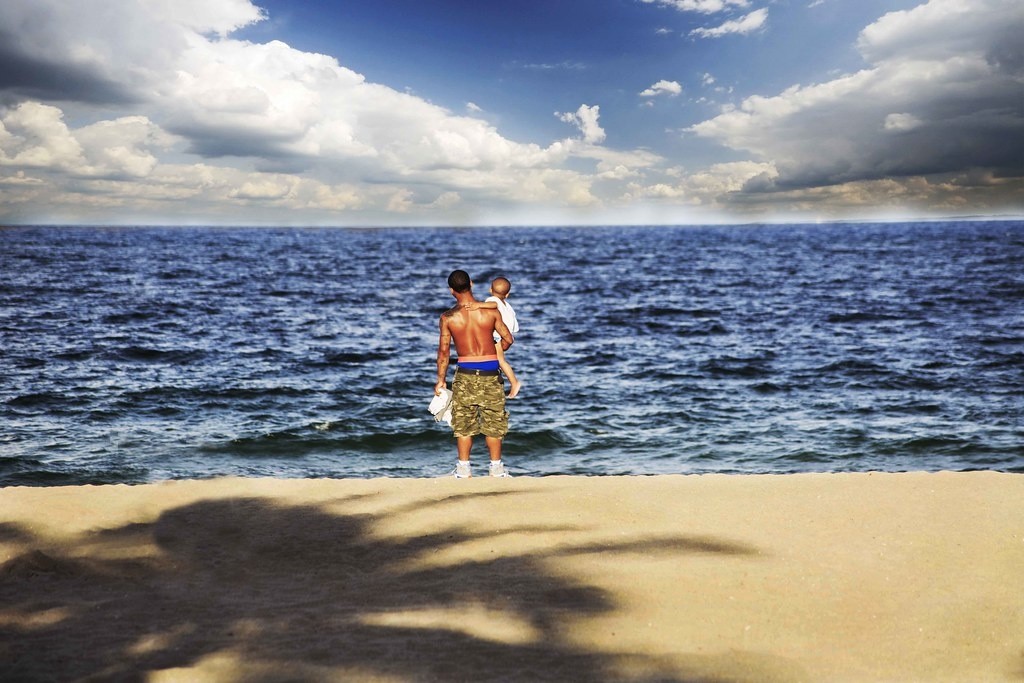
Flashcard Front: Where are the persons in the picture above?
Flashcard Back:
[466,277,521,396]
[434,270,514,479]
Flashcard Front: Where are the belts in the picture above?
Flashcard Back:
[458,368,498,376]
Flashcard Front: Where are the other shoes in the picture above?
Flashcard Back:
[452,464,472,478]
[489,463,512,477]
